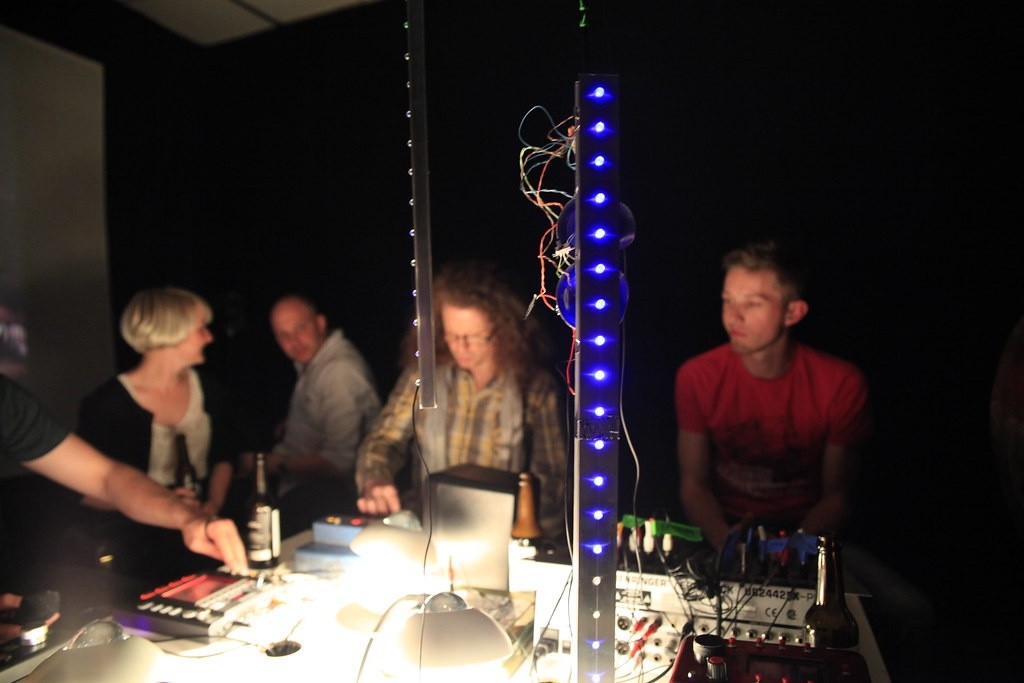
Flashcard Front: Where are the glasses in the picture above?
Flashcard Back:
[443,322,499,346]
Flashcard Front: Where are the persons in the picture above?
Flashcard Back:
[353,264,570,540]
[0,372,248,644]
[78,286,236,613]
[243,296,380,536]
[676,245,934,683]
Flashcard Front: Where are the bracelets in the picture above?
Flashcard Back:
[279,458,288,472]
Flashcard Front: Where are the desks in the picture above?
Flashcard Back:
[1,511,890,683]
